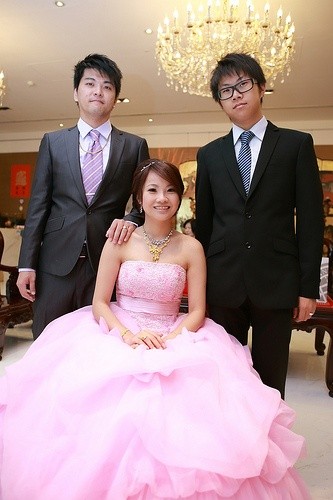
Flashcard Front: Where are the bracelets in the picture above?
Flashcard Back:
[121,329,129,337]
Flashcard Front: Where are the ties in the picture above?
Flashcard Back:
[81,130,103,206]
[237,131,255,196]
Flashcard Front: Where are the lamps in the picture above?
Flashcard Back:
[157,0,298,97]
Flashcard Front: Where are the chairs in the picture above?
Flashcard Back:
[0,227,33,361]
[292,237,333,397]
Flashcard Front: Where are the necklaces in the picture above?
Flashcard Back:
[143,226,174,262]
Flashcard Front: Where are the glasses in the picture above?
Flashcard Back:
[217,77,258,101]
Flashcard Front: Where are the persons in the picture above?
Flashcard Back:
[0,159,310,500]
[16,53,150,341]
[183,219,195,236]
[195,53,325,400]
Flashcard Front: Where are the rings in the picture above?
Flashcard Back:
[309,312,313,315]
[123,225,128,230]
[142,336,147,340]
